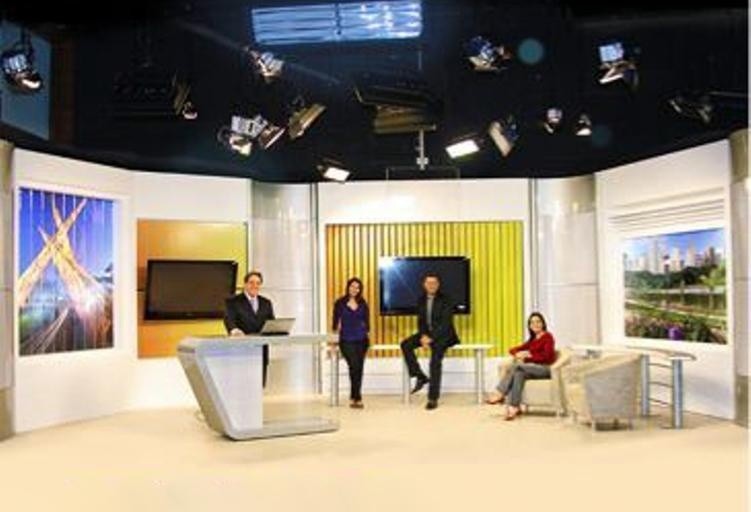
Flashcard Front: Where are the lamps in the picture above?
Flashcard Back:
[444,107,598,161]
[1,26,44,93]
[462,26,513,74]
[217,43,355,185]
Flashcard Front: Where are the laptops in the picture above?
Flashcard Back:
[251,318,294,336]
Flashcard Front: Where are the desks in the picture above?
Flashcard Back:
[324,344,496,408]
[176,333,341,441]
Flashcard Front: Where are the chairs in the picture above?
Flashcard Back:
[561,349,638,433]
[501,346,570,417]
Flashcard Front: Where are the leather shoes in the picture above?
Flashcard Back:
[411,376,429,395]
[426,400,438,409]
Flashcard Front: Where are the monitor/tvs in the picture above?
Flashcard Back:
[145,259,238,319]
[379,256,470,316]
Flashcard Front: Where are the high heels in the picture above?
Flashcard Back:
[504,410,522,420]
[484,394,505,405]
[349,399,357,409]
[358,400,363,408]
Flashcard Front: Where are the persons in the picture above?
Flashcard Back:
[223,271,275,389]
[400,270,462,410]
[485,312,557,420]
[332,276,370,410]
